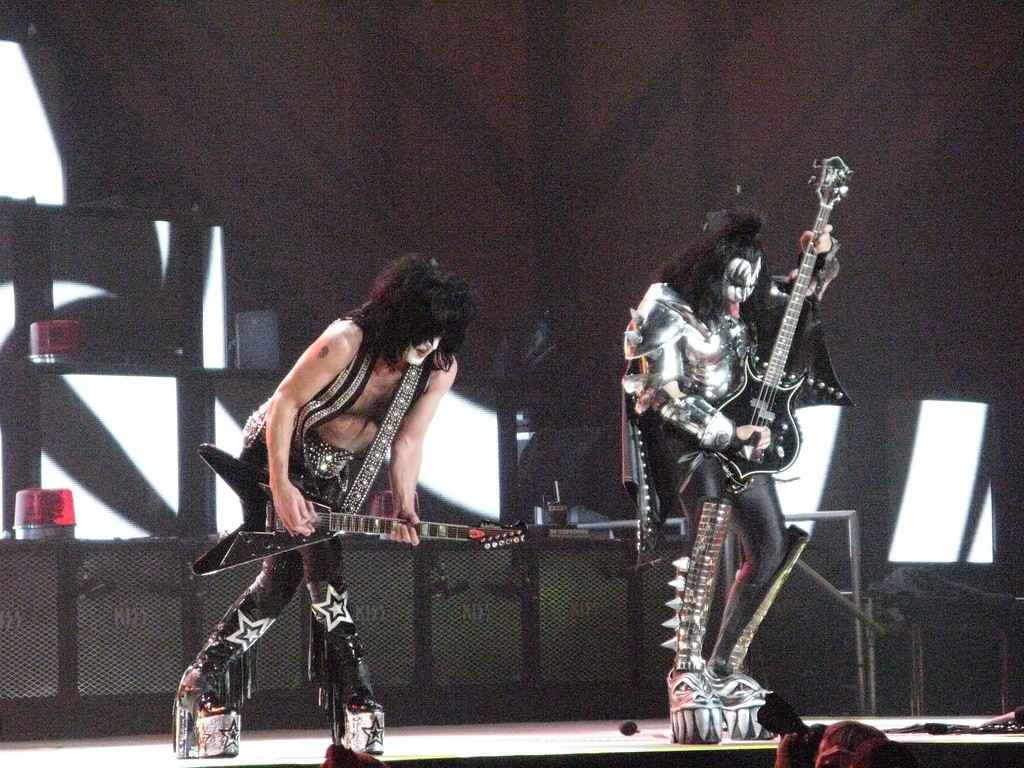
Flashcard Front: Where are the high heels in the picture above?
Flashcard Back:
[172,668,240,760]
[336,681,385,755]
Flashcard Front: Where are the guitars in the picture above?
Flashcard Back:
[703,152,857,483]
[183,438,531,577]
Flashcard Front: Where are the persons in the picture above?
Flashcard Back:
[773,721,919,768]
[171,255,470,759]
[622,208,839,745]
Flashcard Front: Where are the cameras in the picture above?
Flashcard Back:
[757,692,827,768]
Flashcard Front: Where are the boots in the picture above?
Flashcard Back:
[705,524,811,741]
[659,498,733,744]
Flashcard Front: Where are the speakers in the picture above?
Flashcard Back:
[0,529,741,740]
